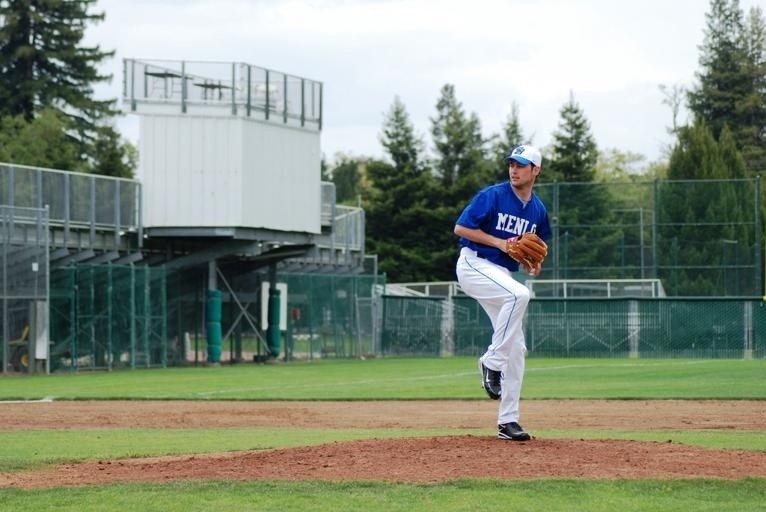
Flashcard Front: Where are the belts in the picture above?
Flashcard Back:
[476,251,487,259]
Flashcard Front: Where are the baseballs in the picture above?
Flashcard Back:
[523,263,535,276]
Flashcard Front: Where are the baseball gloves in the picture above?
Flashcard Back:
[506,232,548,272]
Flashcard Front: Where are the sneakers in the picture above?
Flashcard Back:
[480,357,503,400]
[498,420,531,440]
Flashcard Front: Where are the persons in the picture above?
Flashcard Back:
[451,143,555,444]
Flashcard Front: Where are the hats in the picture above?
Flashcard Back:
[504,144,543,170]
